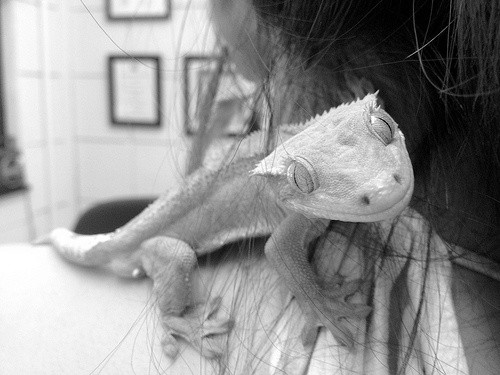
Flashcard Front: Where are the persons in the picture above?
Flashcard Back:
[192,0,498,373]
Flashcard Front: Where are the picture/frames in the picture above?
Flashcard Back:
[108,53,161,127]
[181,54,262,138]
[104,0,174,22]
